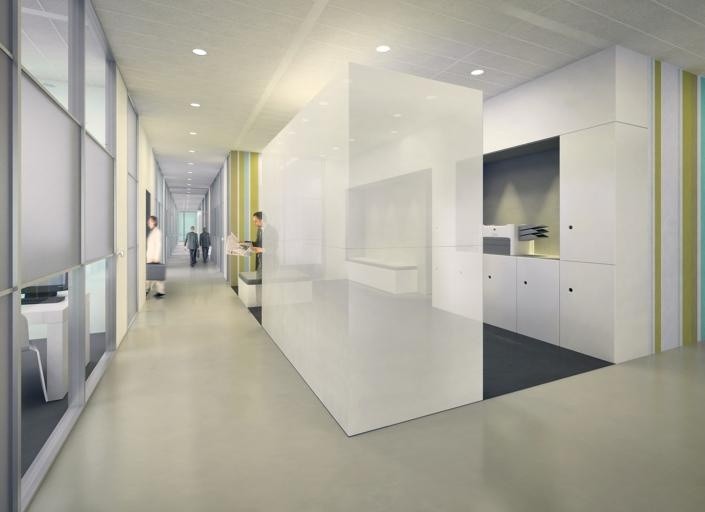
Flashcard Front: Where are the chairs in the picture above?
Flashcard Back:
[20,312,50,403]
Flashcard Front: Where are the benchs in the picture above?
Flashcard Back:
[238,271,314,309]
[348,257,420,296]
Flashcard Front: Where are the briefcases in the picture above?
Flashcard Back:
[146,262,166,281]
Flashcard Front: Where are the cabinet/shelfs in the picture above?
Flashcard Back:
[431,122,655,364]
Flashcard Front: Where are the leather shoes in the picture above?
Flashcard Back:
[154,292,164,297]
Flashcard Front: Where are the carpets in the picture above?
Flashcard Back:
[230,277,615,401]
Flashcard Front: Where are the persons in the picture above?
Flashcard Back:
[183,226,199,267]
[240,212,279,270]
[199,228,211,263]
[146,216,166,296]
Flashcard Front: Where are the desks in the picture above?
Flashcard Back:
[21,288,88,402]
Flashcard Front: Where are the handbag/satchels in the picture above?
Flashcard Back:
[196,248,200,257]
